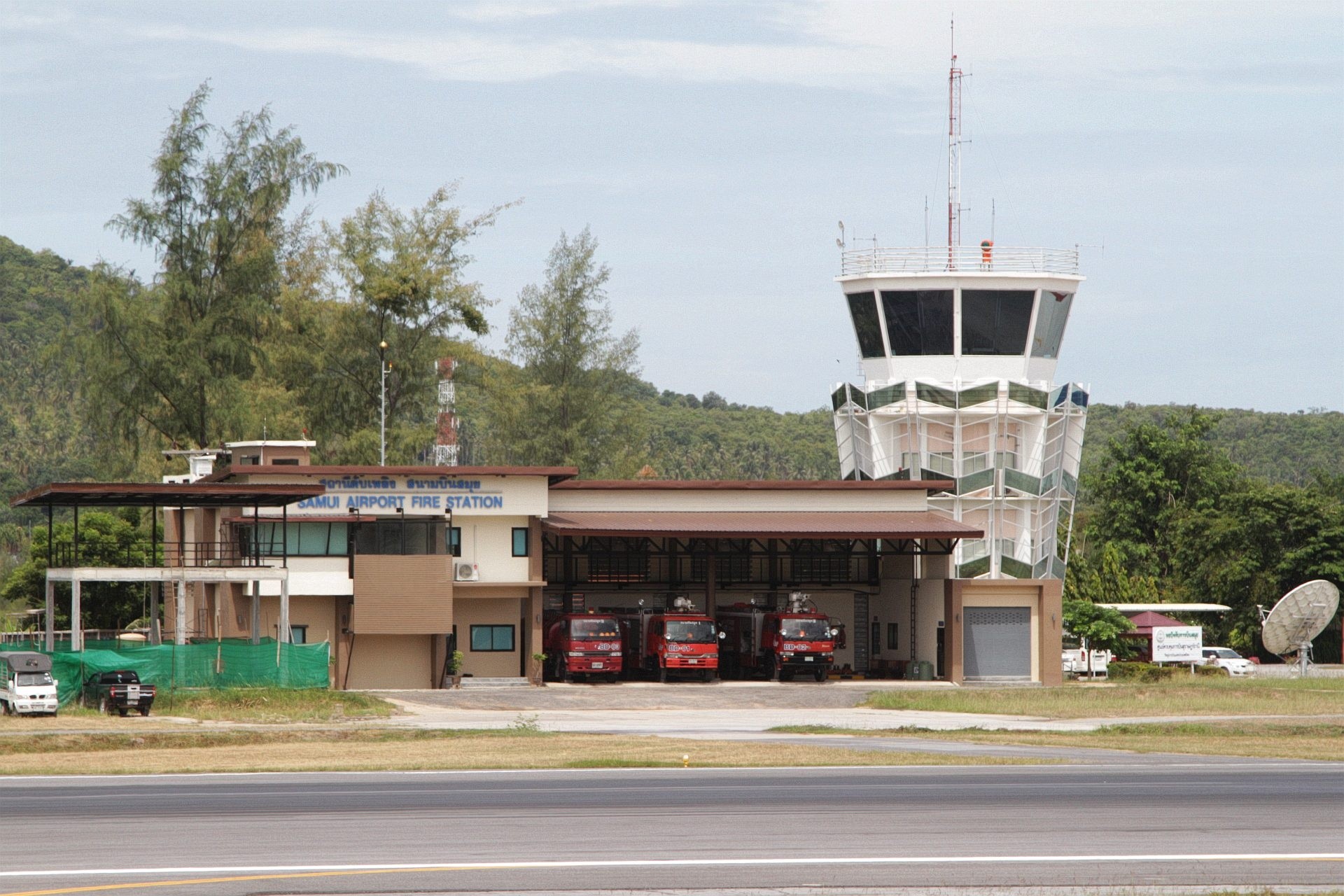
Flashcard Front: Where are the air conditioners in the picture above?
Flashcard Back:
[455,562,479,581]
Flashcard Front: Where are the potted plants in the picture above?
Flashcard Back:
[532,654,548,683]
[448,650,464,685]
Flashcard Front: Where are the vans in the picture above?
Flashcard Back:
[1061,632,1117,673]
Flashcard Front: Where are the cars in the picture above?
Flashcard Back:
[1185,646,1260,678]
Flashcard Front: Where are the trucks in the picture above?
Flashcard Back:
[0,652,60,719]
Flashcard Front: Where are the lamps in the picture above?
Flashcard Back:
[446,508,452,521]
[349,507,359,520]
[396,508,404,519]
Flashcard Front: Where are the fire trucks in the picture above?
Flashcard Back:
[598,597,726,682]
[543,605,622,683]
[712,591,844,682]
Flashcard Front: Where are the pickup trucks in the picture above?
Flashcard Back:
[83,670,156,718]
